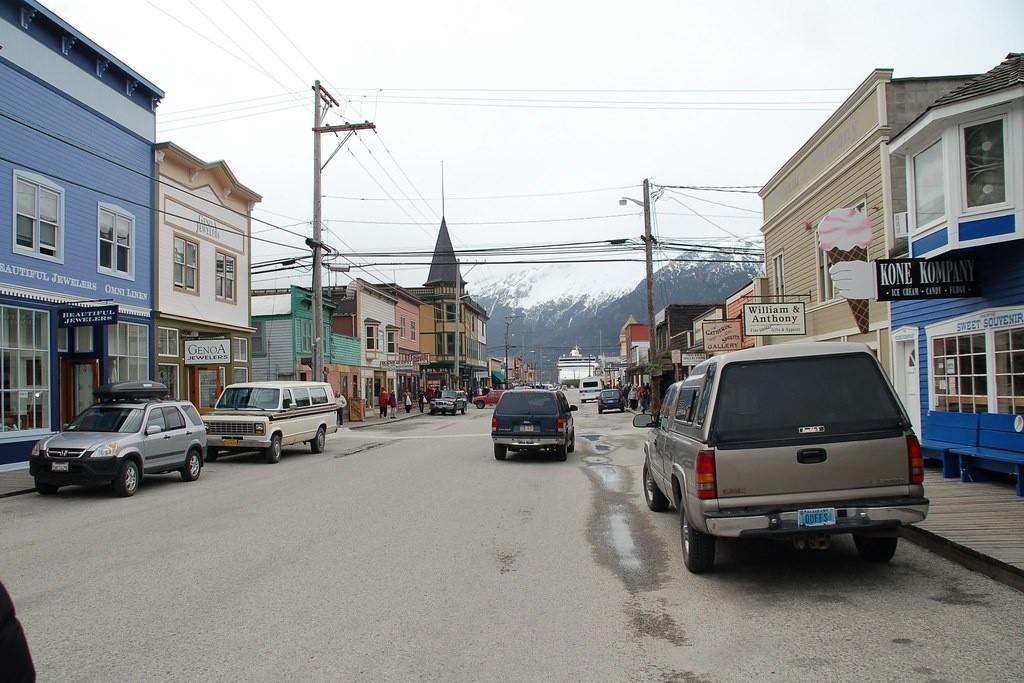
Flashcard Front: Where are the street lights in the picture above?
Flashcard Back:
[609,238,660,424]
[453,293,480,388]
[522,350,535,385]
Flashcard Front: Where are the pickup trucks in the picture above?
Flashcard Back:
[631,338,931,573]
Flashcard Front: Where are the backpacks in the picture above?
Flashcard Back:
[641,392,647,400]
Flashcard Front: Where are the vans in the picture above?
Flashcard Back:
[491,388,577,461]
[579,377,605,403]
[201,380,338,463]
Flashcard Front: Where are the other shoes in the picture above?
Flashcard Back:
[390,417,396,419]
[340,425,343,427]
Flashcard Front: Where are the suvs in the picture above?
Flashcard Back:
[29,380,209,496]
[473,389,508,410]
[429,390,468,415]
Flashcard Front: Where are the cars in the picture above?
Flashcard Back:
[595,388,625,414]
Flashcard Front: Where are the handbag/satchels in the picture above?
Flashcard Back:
[406,395,411,405]
[423,396,427,403]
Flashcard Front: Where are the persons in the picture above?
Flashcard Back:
[622,382,650,414]
[461,386,482,403]
[403,389,412,413]
[482,386,493,396]
[335,392,347,428]
[425,385,440,404]
[605,383,612,389]
[418,388,426,413]
[378,386,389,419]
[388,391,397,419]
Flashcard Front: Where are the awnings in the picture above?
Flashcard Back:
[491,371,512,384]
